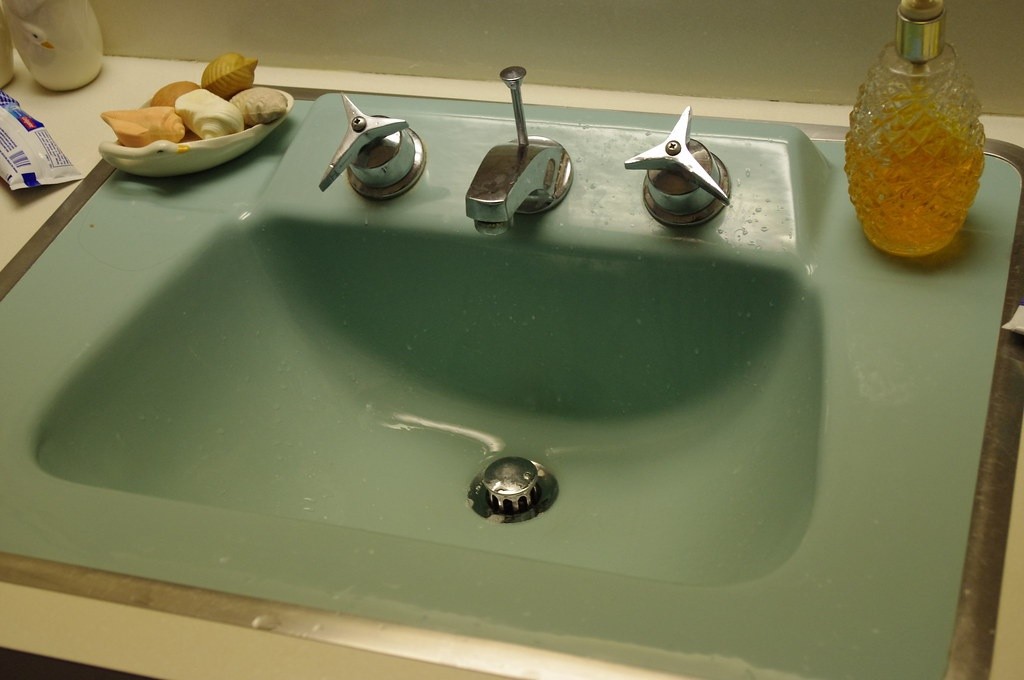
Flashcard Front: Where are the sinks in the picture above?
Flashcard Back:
[30,205,841,593]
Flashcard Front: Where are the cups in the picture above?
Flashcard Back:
[0,9,14,89]
[0,0,103,90]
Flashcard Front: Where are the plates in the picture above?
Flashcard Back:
[98,90,295,175]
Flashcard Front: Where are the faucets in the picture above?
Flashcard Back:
[464,64,574,237]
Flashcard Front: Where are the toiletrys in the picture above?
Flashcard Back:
[842,0,984,262]
[0,87,86,197]
